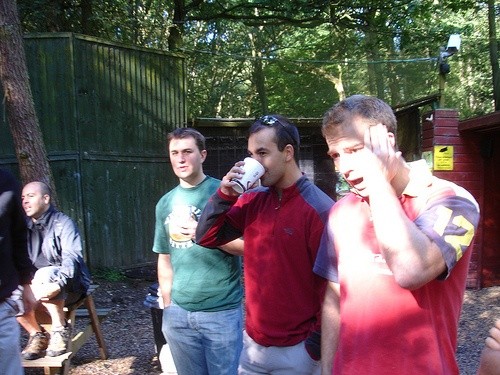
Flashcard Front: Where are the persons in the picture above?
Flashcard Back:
[153,127,244,375]
[10,181,90,361]
[311,94,481,375]
[195,113,338,375]
[0,170,37,375]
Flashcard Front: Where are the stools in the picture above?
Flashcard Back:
[23,285,108,375]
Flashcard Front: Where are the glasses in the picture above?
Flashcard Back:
[254,115,298,144]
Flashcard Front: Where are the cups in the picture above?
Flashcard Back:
[229,156,266,193]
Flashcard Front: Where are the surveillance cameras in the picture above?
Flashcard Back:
[447,35,461,52]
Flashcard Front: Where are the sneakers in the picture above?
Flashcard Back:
[46,317,71,357]
[21,326,50,359]
